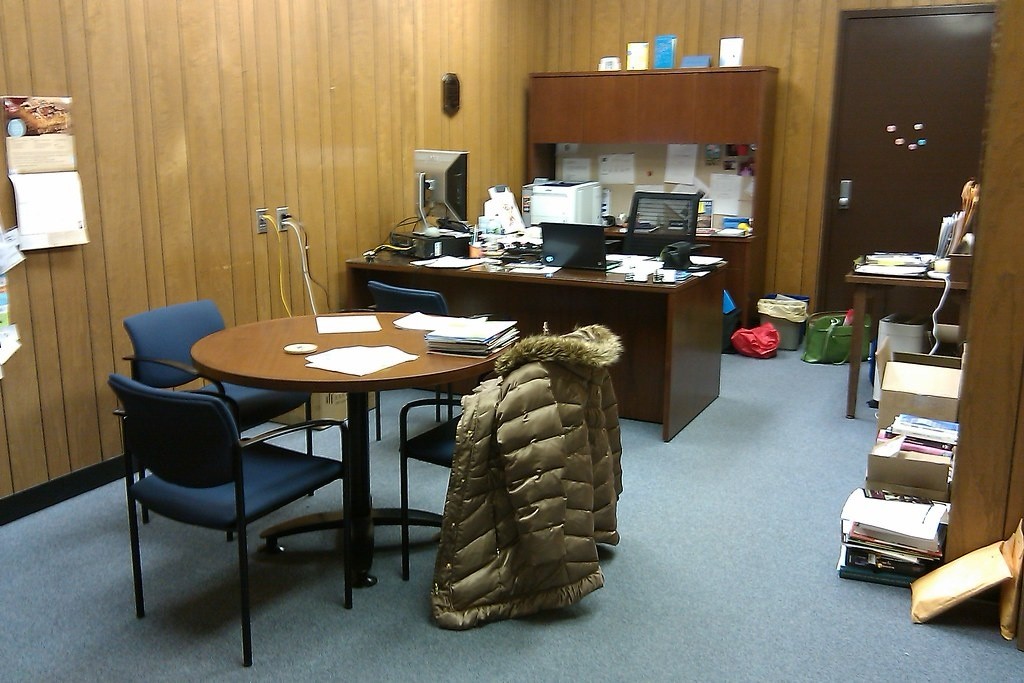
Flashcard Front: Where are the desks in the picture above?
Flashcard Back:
[345,238,728,442]
[844,270,971,419]
[191,312,513,589]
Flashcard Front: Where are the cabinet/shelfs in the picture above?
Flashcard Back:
[526,65,780,329]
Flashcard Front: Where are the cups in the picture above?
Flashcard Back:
[468,242,483,258]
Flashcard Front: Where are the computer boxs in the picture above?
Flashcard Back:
[391,232,473,259]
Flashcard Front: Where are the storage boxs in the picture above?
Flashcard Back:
[865,453,950,502]
[874,336,961,434]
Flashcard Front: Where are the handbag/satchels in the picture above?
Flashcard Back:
[730,322,780,358]
[800,310,871,365]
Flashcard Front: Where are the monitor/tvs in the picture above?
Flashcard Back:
[411,148,472,238]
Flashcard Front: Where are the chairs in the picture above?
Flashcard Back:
[622,191,700,256]
[368,280,448,317]
[121,299,315,497]
[399,324,604,580]
[105,372,355,667]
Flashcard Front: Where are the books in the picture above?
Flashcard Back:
[835,415,960,589]
[427,321,521,358]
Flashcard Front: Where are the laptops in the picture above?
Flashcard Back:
[541,222,623,270]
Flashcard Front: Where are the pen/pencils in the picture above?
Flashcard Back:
[472,224,477,244]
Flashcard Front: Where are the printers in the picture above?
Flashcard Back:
[531,181,603,225]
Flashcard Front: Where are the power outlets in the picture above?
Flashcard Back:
[257,208,268,234]
[277,207,289,233]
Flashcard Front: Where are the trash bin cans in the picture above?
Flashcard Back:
[756,298,807,351]
[764,294,810,344]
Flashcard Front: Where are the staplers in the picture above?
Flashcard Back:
[738,223,754,237]
